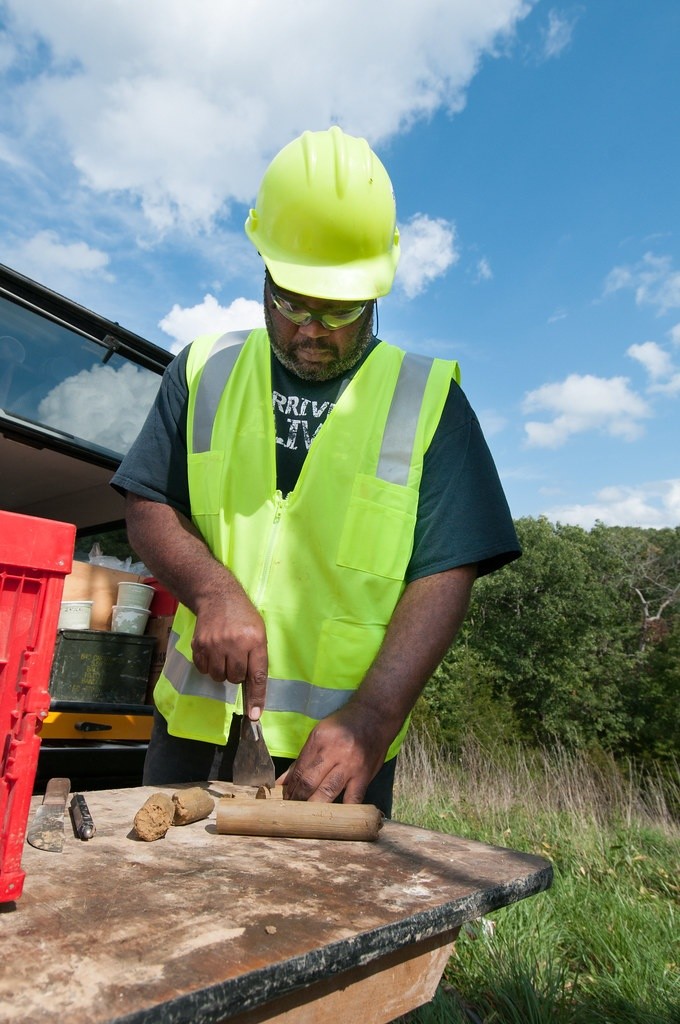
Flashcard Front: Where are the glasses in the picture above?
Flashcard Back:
[265,271,369,329]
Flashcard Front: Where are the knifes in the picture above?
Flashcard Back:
[28,777,72,850]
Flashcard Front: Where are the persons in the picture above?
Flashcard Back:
[109,127,524,820]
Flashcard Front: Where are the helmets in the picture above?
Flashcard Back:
[243,125,402,300]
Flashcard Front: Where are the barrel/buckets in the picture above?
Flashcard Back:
[142,579,179,617]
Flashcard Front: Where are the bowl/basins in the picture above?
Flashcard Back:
[117,582,157,610]
[58,601,94,630]
[111,605,151,635]
